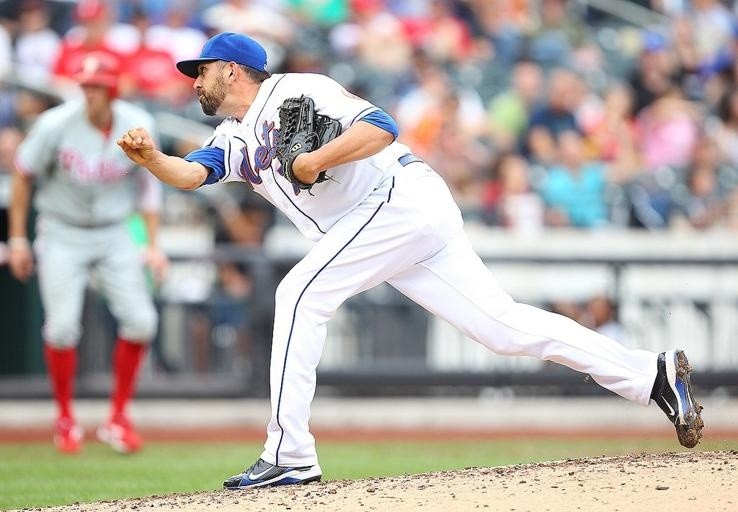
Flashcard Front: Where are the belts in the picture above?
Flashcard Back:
[399,154,423,167]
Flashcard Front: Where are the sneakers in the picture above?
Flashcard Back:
[223,458,323,491]
[655,349,703,449]
[54,417,143,454]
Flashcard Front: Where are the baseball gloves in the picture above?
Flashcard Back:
[273,94,343,194]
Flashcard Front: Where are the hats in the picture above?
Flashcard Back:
[176,31,268,80]
[75,52,120,87]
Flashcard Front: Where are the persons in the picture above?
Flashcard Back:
[116,32,705,492]
[8,51,170,455]
